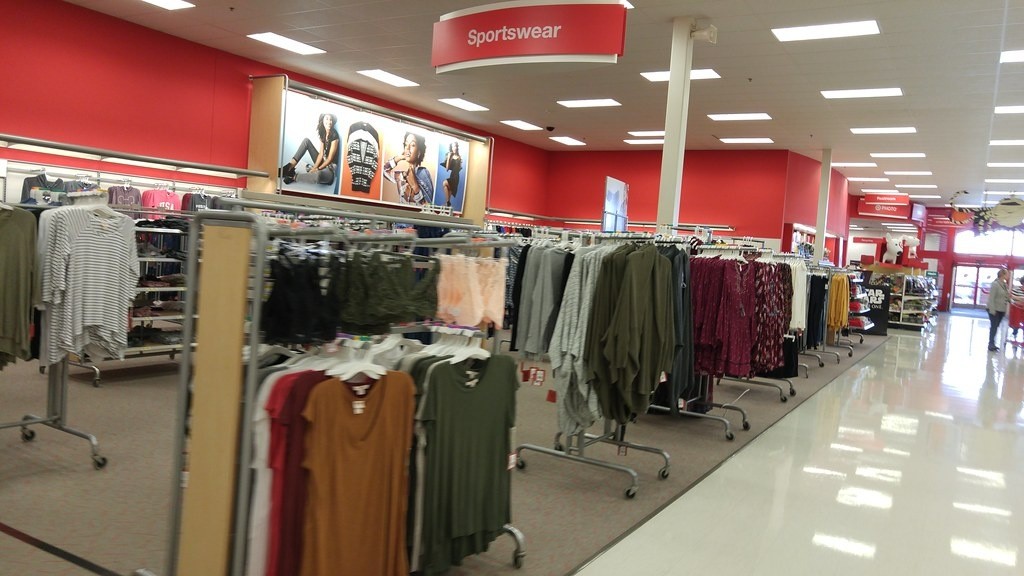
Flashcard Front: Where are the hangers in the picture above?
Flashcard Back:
[490,222,849,275]
[261,327,493,381]
[31,166,234,196]
[0,198,126,222]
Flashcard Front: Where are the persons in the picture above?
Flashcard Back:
[959,274,970,303]
[278,113,340,186]
[440,142,463,210]
[1009,277,1024,338]
[988,269,1011,351]
[383,131,434,206]
[618,184,628,231]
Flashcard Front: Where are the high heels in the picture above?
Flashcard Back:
[306,164,313,172]
[278,163,296,184]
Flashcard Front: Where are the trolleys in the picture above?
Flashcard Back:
[1006,300,1024,354]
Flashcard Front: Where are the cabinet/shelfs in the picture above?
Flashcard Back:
[36,229,198,374]
[844,267,938,337]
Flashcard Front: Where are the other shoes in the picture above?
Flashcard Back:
[988,345,999,352]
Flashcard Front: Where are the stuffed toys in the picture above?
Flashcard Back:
[948,190,1024,236]
[882,233,921,264]
